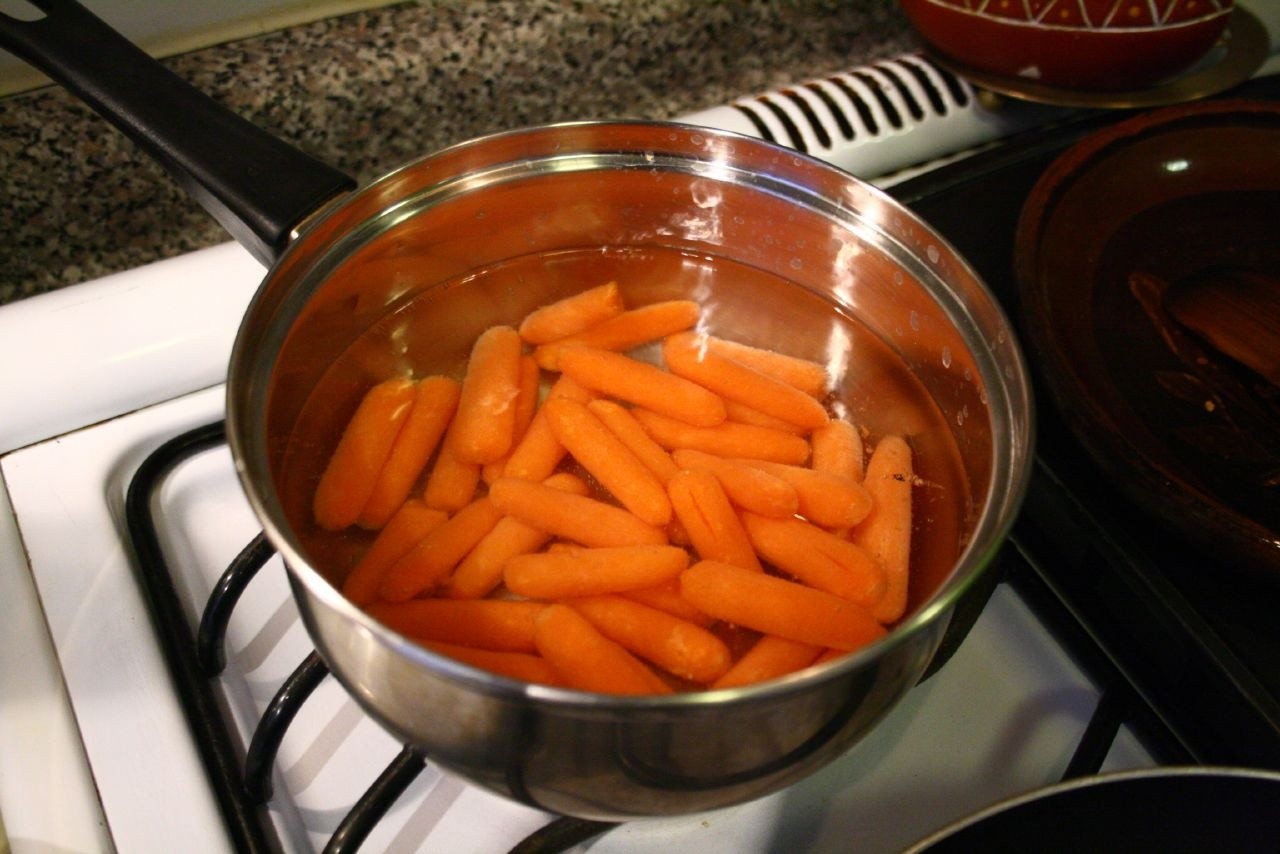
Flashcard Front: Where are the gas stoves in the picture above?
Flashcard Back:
[2,55,1280,853]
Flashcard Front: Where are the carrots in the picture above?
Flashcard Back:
[307,283,912,698]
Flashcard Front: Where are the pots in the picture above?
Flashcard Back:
[896,764,1280,853]
[1015,96,1280,578]
[0,1,1033,822]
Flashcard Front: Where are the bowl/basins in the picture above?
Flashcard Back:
[901,0,1238,89]
